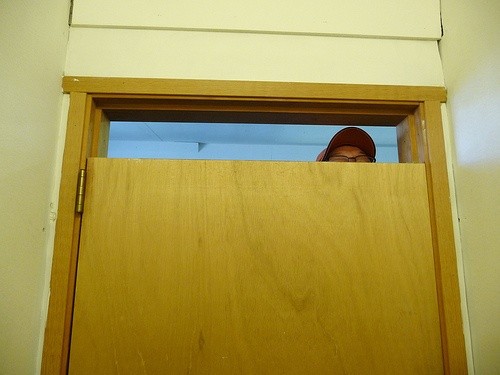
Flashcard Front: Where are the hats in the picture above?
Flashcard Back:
[316,126,376,162]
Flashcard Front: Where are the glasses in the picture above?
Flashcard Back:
[325,154,376,163]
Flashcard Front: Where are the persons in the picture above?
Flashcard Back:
[316,127,377,163]
